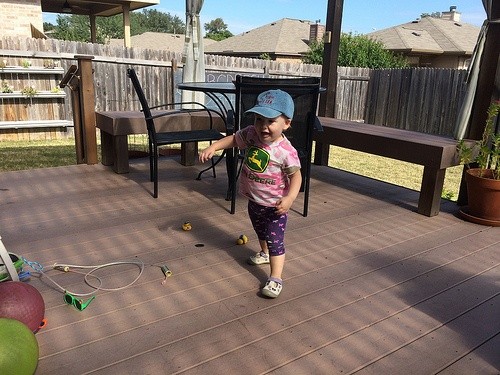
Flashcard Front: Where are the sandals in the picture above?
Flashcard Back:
[261,274,283,297]
[247,250,270,264]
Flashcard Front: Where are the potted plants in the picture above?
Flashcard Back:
[455,103,500,227]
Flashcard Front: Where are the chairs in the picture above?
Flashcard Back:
[127,68,238,201]
[226,75,320,217]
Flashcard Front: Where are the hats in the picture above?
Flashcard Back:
[245,89,295,119]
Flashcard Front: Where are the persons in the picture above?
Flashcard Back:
[198,89,303,298]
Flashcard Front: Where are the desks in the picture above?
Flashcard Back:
[176,82,326,201]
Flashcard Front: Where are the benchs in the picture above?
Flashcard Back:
[94,109,234,175]
[311,116,481,217]
